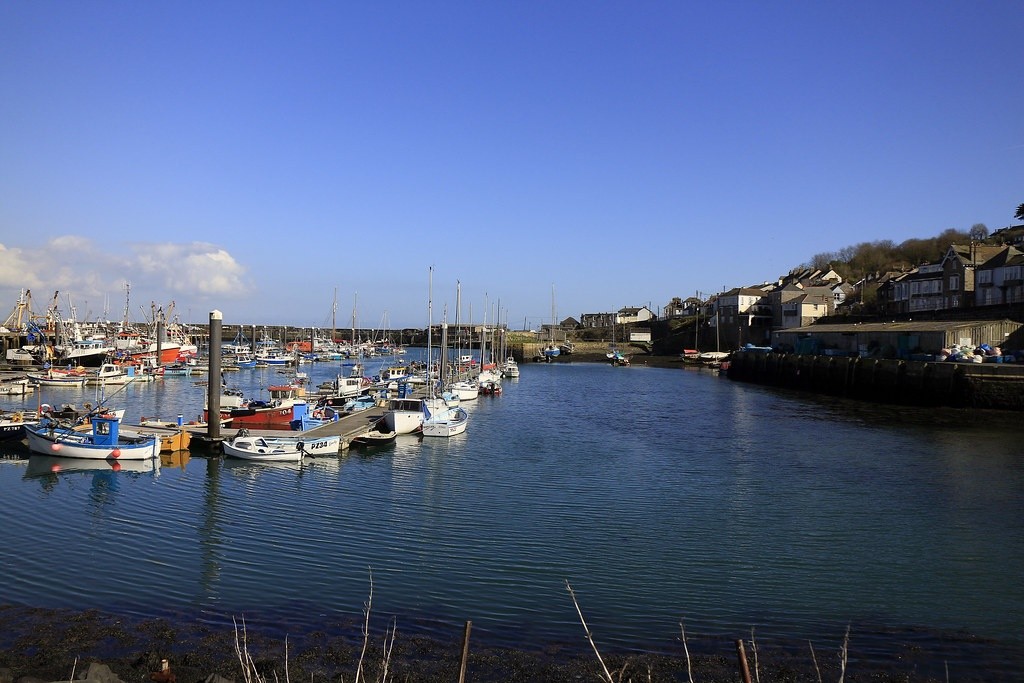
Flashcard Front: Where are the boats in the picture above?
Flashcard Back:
[680,348,730,365]
[605,350,631,367]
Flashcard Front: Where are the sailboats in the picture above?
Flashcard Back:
[1,264,526,470]
[533,281,575,363]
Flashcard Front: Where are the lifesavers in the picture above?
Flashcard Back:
[313,408,324,417]
[98,414,111,417]
[361,377,369,384]
[39,403,51,415]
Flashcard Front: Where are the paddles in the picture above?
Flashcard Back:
[298,446,315,458]
